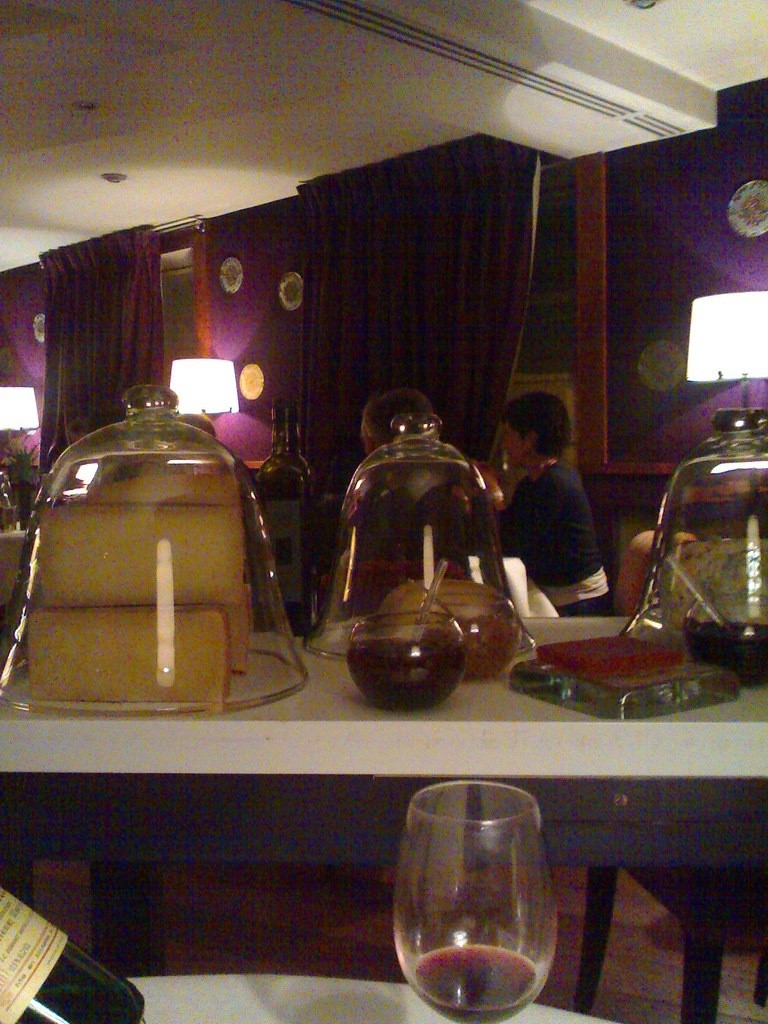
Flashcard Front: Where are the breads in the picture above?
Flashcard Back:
[380,577,520,679]
[0,471,253,704]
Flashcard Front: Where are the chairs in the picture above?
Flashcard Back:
[572,865,768,1022]
[613,531,655,615]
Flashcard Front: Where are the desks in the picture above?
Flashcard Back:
[1,615,768,1024]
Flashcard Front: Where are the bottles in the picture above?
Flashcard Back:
[0,471,16,532]
[0,387,307,717]
[308,414,540,665]
[618,408,766,688]
[247,398,320,637]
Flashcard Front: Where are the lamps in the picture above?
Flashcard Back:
[0,384,39,459]
[168,358,240,420]
[684,292,768,409]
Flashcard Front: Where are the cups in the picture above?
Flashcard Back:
[347,582,521,713]
[391,776,557,1023]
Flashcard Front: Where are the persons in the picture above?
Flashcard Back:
[461,390,613,617]
[312,386,495,579]
